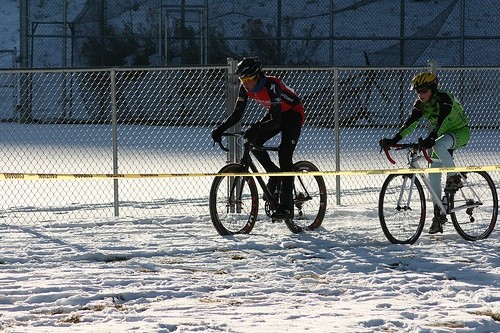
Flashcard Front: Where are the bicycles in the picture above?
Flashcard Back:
[379,136,499,246]
[208,132,327,236]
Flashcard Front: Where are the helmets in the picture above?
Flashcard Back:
[411,73,438,89]
[235,57,262,79]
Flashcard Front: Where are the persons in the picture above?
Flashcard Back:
[378,72,472,233]
[210,57,304,220]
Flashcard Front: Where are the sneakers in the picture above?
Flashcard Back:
[263,178,279,200]
[271,206,294,218]
[429,215,447,234]
[444,177,464,192]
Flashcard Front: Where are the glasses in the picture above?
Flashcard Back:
[240,75,256,83]
[417,83,435,94]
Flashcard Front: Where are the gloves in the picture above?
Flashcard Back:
[212,127,224,141]
[379,133,403,150]
[422,131,439,148]
[244,128,257,140]
